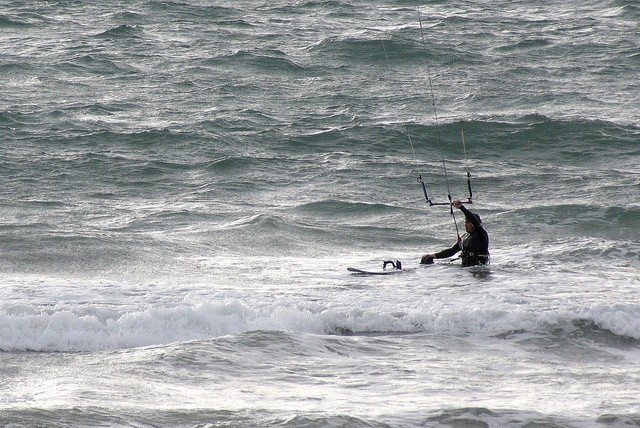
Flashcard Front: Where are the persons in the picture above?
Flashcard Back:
[423,199,489,269]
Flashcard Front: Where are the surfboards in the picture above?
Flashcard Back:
[348,262,419,274]
